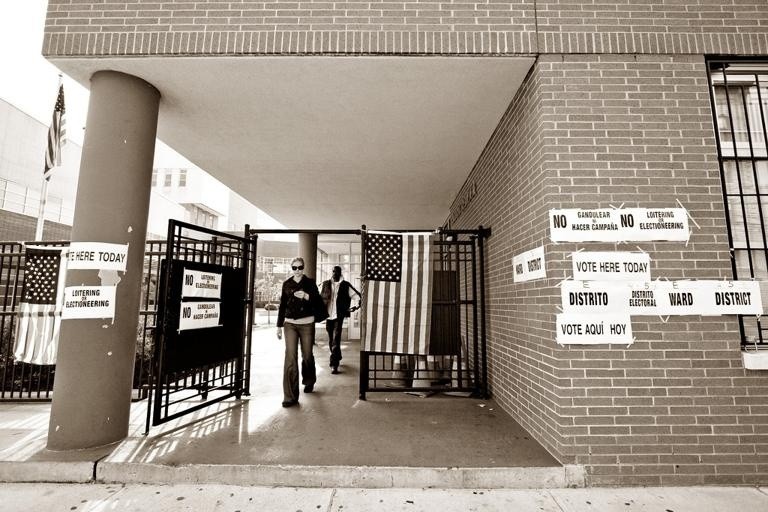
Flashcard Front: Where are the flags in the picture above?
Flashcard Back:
[11,242,69,367]
[42,80,68,185]
[360,230,434,356]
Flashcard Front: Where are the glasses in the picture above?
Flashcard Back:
[292,266,304,270]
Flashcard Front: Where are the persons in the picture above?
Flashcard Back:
[316,265,361,375]
[276,257,316,407]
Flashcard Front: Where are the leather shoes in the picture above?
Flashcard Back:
[331,366,338,374]
[283,402,297,406]
[304,384,313,393]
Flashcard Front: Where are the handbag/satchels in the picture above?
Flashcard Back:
[314,295,329,322]
